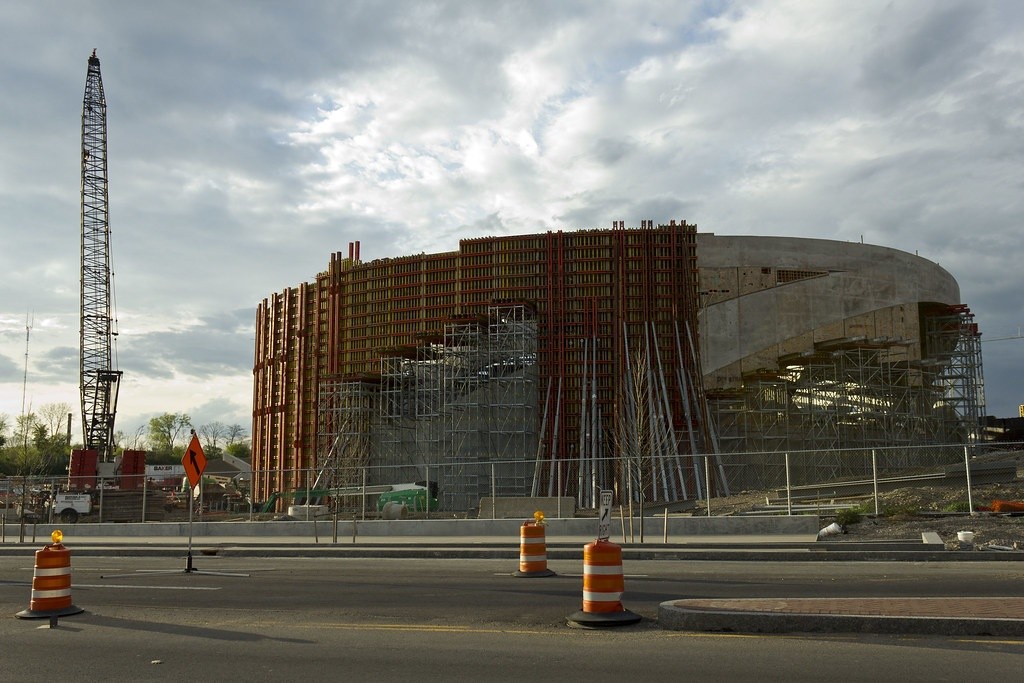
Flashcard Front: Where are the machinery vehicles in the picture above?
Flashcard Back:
[263,481,438,515]
[15,45,202,526]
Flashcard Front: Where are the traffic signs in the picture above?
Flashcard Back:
[598,489,614,540]
